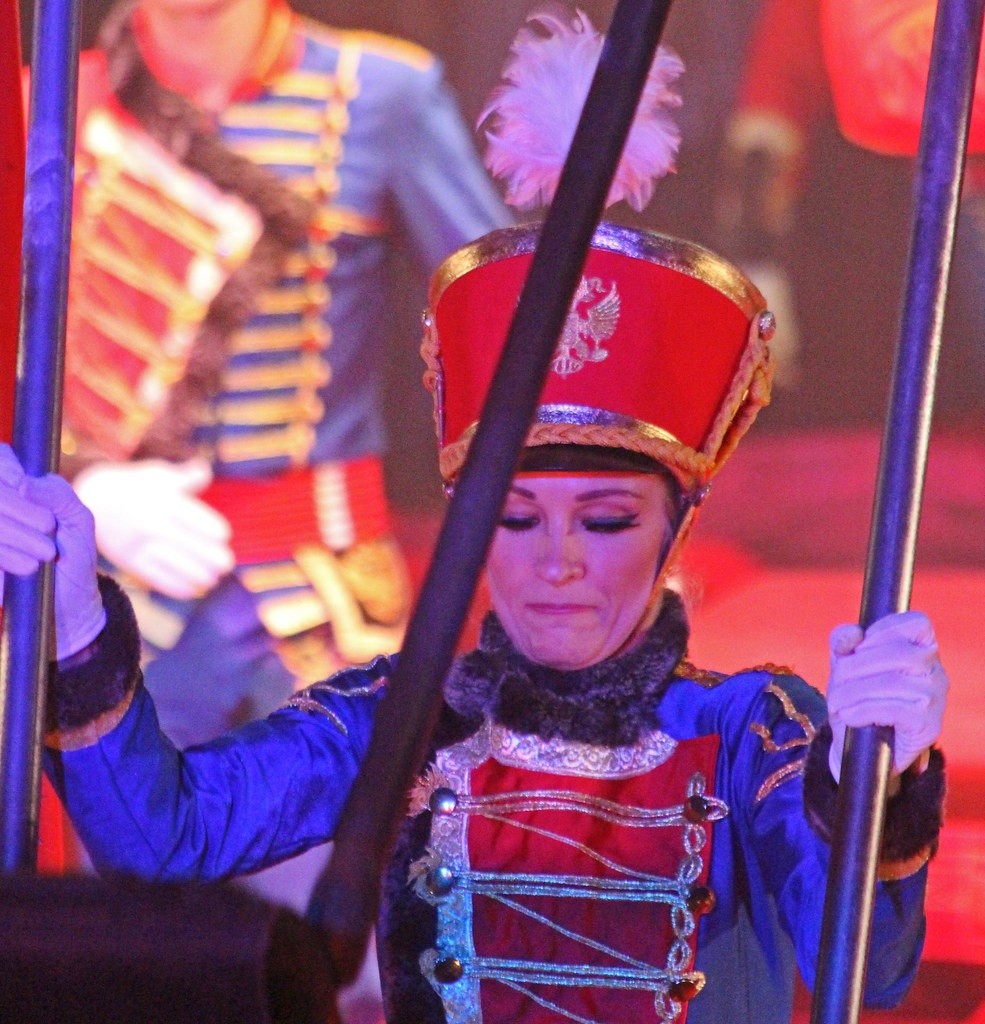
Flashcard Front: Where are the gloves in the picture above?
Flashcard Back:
[826,610,949,787]
[0,443,108,669]
[68,451,238,607]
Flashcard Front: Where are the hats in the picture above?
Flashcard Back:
[421,4,775,504]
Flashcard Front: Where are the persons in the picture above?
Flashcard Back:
[0,208,948,1021]
[0,0,545,1024]
